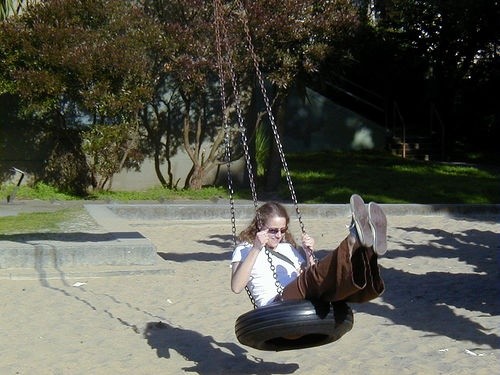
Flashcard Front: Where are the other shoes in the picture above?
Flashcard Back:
[367,201,389,256]
[345,194,374,248]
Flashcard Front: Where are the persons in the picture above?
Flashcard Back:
[230,193,389,340]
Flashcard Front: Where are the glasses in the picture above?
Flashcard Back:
[262,224,288,235]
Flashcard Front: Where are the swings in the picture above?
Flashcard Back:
[214,0,356,350]
